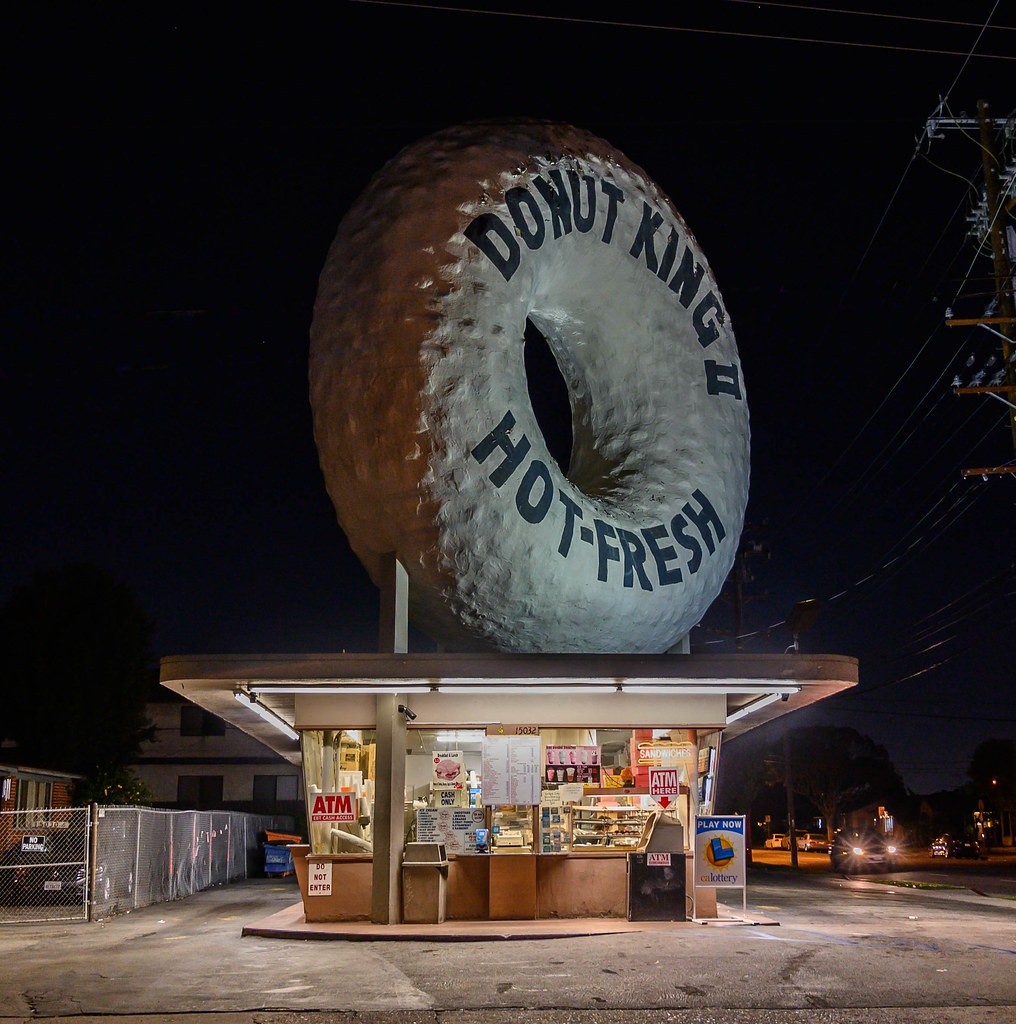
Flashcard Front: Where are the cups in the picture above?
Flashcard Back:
[340,778,372,817]
[545,745,600,784]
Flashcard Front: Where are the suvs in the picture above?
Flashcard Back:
[781,829,809,850]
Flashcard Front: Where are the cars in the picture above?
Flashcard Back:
[763,833,786,848]
[2,833,137,906]
[828,826,898,874]
[797,833,829,852]
[927,832,982,860]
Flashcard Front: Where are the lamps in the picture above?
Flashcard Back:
[232,688,300,742]
[620,686,803,694]
[435,686,620,693]
[726,693,782,726]
[251,686,433,694]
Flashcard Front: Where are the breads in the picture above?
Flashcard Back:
[309,109,752,652]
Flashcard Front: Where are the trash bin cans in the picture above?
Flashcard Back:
[624,853,688,921]
[262,840,295,877]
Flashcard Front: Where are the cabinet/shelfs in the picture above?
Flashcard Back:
[570,785,692,851]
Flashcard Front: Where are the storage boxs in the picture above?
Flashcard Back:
[602,775,621,788]
[494,831,523,846]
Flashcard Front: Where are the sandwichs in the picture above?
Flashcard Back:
[435,760,460,782]
